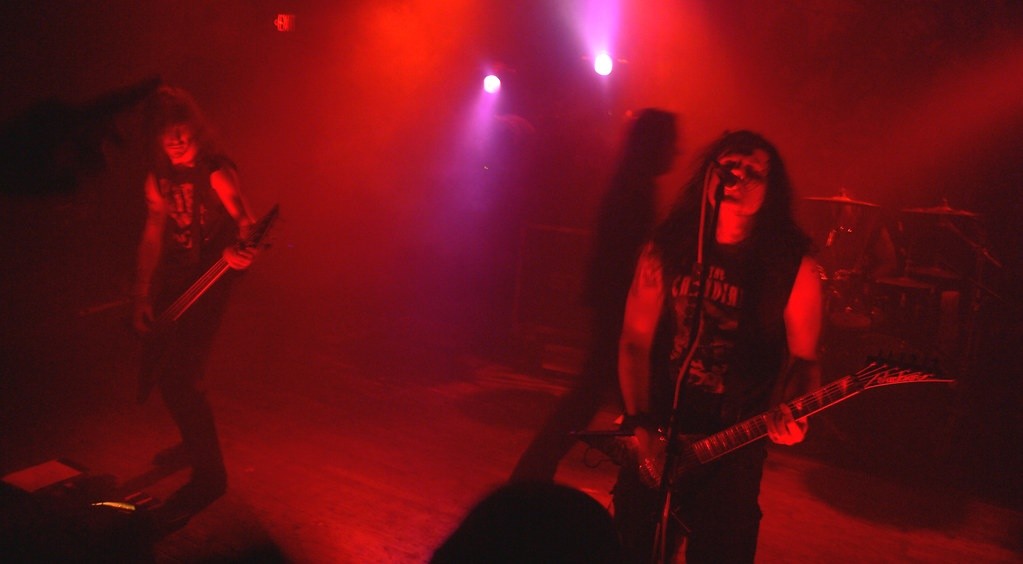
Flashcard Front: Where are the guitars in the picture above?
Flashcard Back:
[108,205,282,406]
[569,359,958,549]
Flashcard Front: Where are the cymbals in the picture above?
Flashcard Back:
[799,193,880,209]
[901,205,973,217]
[909,267,967,280]
[877,273,939,289]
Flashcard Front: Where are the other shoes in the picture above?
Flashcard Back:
[156,441,194,471]
[163,482,227,512]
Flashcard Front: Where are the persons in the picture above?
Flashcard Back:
[580,106,681,381]
[615,127,827,564]
[126,84,276,525]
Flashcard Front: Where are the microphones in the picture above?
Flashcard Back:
[712,161,747,201]
